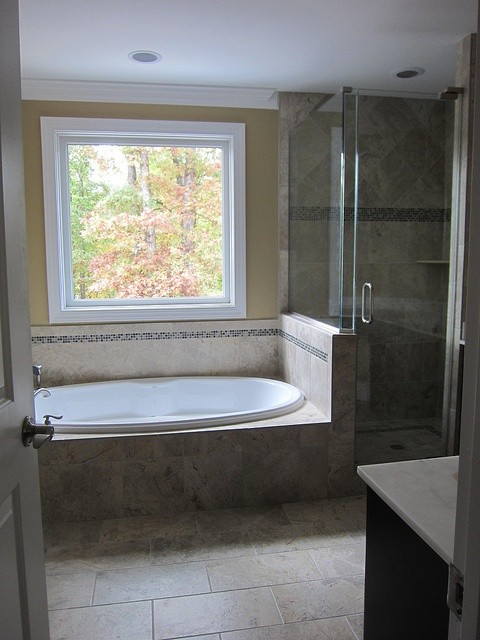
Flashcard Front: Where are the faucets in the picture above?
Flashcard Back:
[33,388,51,397]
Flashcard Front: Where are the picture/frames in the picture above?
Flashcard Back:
[40,116,247,325]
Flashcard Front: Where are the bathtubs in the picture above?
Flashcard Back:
[33,375,304,433]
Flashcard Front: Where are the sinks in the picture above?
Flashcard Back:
[427,468,459,505]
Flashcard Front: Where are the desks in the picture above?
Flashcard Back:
[357,455,460,639]
